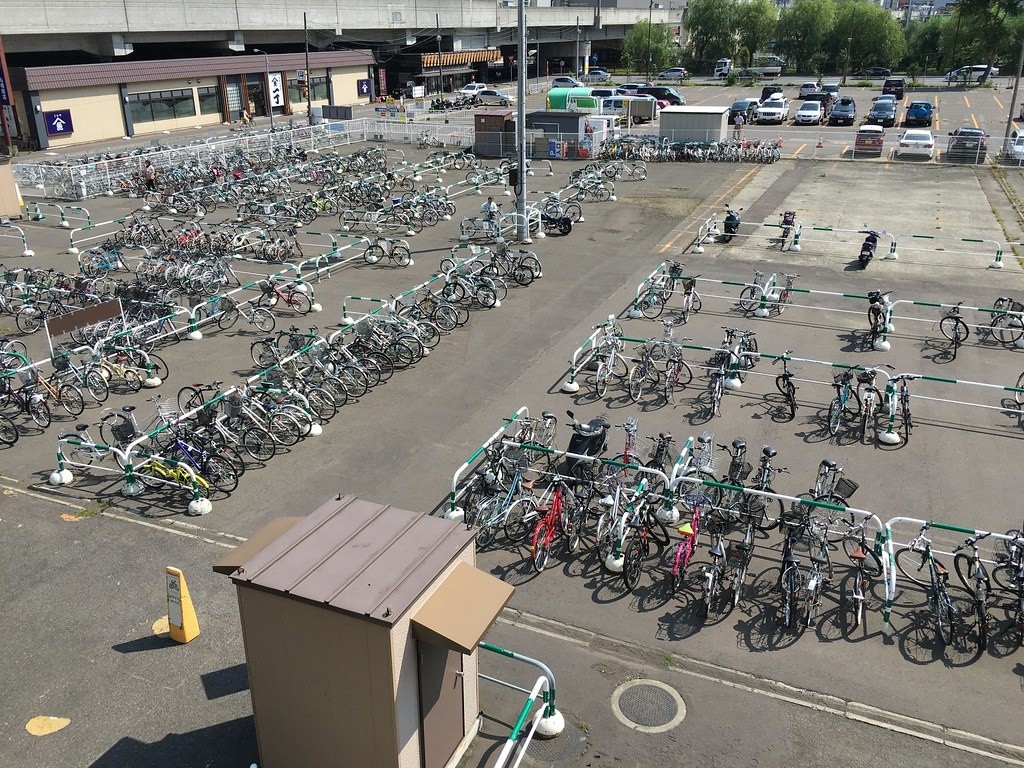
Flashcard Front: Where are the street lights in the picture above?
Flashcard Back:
[252,46,273,132]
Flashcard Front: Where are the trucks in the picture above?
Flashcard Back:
[713,56,764,77]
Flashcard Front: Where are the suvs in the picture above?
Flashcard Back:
[906,101,936,127]
[761,86,783,100]
[475,89,517,106]
[754,93,789,124]
[460,83,488,99]
[657,68,689,80]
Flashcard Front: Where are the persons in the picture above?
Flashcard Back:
[145,161,156,190]
[480,198,498,235]
[732,112,745,139]
[241,107,250,127]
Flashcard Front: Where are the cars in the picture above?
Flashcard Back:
[580,71,612,83]
[1019,102,1024,121]
[854,66,891,77]
[854,125,885,156]
[744,97,764,108]
[795,100,825,125]
[976,65,1000,77]
[945,65,990,82]
[591,82,670,123]
[829,96,857,126]
[897,129,938,160]
[1007,128,1024,166]
[867,93,900,127]
[727,101,755,126]
[552,77,586,90]
[947,127,990,163]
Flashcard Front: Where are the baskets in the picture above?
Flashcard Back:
[0,120,1024,571]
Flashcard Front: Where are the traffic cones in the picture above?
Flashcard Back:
[778,133,784,147]
[742,135,747,148]
[816,135,824,148]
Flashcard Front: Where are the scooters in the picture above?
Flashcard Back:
[857,223,887,269]
[720,203,744,245]
[427,95,479,113]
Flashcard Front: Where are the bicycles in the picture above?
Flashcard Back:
[1014,373,1023,407]
[0,124,1024,654]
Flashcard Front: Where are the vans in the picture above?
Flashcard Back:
[755,55,787,69]
[798,80,841,117]
[637,85,687,106]
[882,77,907,100]
